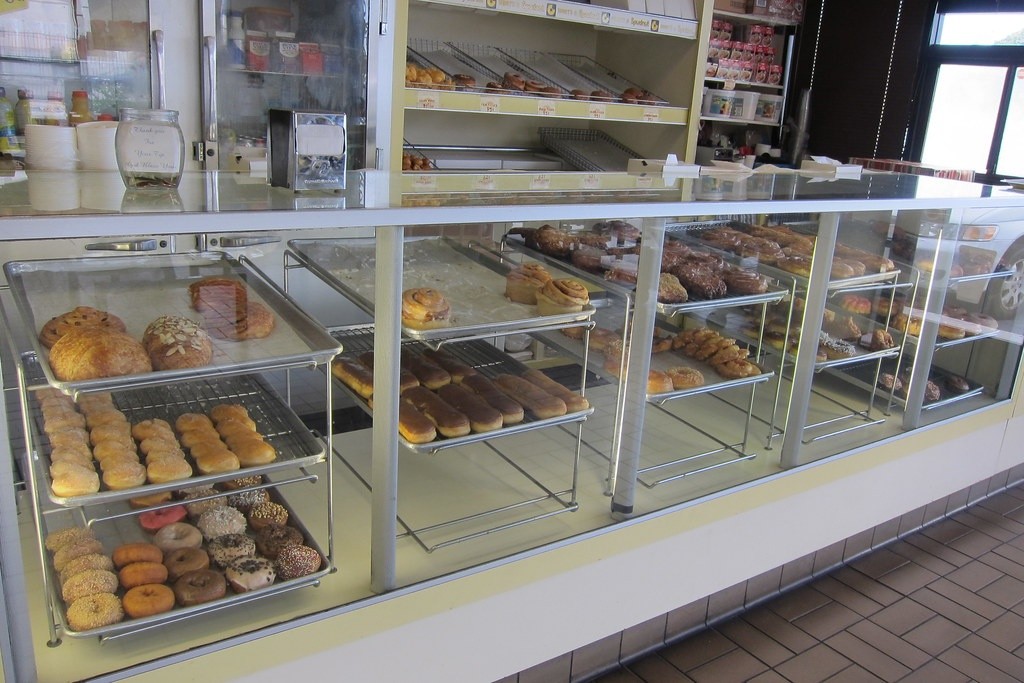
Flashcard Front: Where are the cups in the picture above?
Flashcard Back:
[744,155,756,168]
[770,149,782,157]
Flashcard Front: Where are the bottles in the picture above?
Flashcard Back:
[69,91,92,126]
[14,90,42,150]
[0,87,20,151]
[228,10,246,69]
[45,93,68,126]
[98,114,112,121]
[77,20,150,59]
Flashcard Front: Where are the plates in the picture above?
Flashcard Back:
[24,124,76,170]
[77,121,119,170]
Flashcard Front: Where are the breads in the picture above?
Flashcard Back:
[402,152,430,169]
[526,218,997,302]
[404,63,457,91]
[330,262,590,443]
[38,277,274,499]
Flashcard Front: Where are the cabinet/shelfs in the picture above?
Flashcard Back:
[1,215,1017,647]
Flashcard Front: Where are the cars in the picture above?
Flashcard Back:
[879,195,1024,319]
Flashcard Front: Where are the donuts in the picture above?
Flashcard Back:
[449,71,659,105]
[41,473,322,632]
[563,294,998,404]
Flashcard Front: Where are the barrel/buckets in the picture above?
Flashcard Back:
[755,94,785,124]
[756,144,771,156]
[703,89,736,118]
[729,91,760,120]
